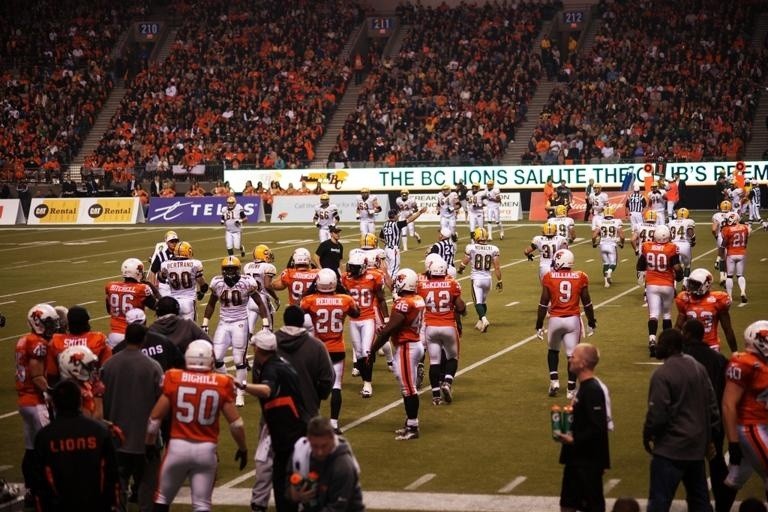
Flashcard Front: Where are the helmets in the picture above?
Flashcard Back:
[316,268,337,293]
[425,253,442,274]
[185,339,214,372]
[59,345,98,380]
[554,205,566,218]
[676,207,689,218]
[472,182,480,194]
[430,259,447,276]
[290,248,311,267]
[346,248,368,279]
[226,197,236,210]
[54,305,69,331]
[603,207,614,218]
[487,180,494,191]
[550,248,575,271]
[633,185,640,192]
[26,304,60,335]
[173,240,192,259]
[653,225,671,243]
[726,213,741,226]
[442,185,451,197]
[221,256,242,286]
[644,210,657,222]
[720,200,732,213]
[650,182,659,191]
[543,222,558,237]
[473,228,488,245]
[592,183,601,195]
[360,233,377,250]
[395,269,417,295]
[400,189,409,202]
[743,320,768,361]
[360,187,369,201]
[320,194,330,208]
[686,268,713,297]
[121,258,143,283]
[253,245,274,263]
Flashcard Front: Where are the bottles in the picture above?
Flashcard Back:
[306,472,317,505]
[551,406,561,441]
[291,474,305,492]
[563,406,581,437]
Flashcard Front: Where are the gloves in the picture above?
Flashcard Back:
[715,255,721,270]
[366,349,374,370]
[527,253,534,262]
[201,319,210,335]
[447,206,455,213]
[458,263,466,275]
[635,248,640,257]
[143,443,158,462]
[689,234,696,248]
[536,323,545,342]
[728,441,744,465]
[593,242,598,248]
[235,447,247,470]
[586,321,597,336]
[495,281,504,296]
[618,242,624,248]
[197,287,205,301]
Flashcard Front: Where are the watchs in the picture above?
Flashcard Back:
[241,379,246,390]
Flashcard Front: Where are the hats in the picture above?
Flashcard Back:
[155,296,179,316]
[165,231,179,242]
[125,309,146,325]
[330,227,342,233]
[437,227,451,237]
[248,329,277,351]
[283,305,304,328]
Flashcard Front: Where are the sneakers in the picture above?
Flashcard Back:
[649,338,657,358]
[361,381,373,397]
[395,427,412,434]
[604,271,612,285]
[475,321,482,330]
[242,246,246,258]
[481,319,490,334]
[740,290,748,303]
[441,383,453,402]
[395,431,420,441]
[352,368,359,377]
[419,364,424,388]
[547,379,559,395]
[235,391,243,409]
[432,396,443,407]
[331,421,343,437]
[566,388,577,400]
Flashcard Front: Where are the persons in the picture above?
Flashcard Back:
[481,180,504,242]
[148,296,214,356]
[663,178,679,222]
[355,187,382,235]
[588,183,609,233]
[313,228,344,283]
[326,0,768,168]
[453,180,469,224]
[144,339,249,512]
[415,253,463,389]
[466,183,487,240]
[21,381,128,512]
[395,188,421,254]
[348,233,386,269]
[436,184,461,243]
[717,217,727,287]
[14,303,61,512]
[733,161,746,188]
[636,226,685,358]
[715,176,727,209]
[545,192,568,218]
[47,307,112,420]
[729,183,743,212]
[379,206,427,281]
[1,1,324,197]
[155,241,209,324]
[551,343,611,512]
[681,320,734,512]
[591,206,625,288]
[535,248,596,400]
[741,180,752,214]
[524,222,568,319]
[667,207,696,299]
[55,306,69,334]
[417,258,468,406]
[148,231,180,286]
[719,170,729,187]
[748,183,767,229]
[201,255,269,409]
[244,244,280,342]
[226,329,309,512]
[721,214,748,304]
[252,305,336,511]
[282,415,366,512]
[104,257,163,349]
[103,322,164,512]
[716,318,768,512]
[625,186,647,236]
[455,229,503,333]
[712,200,740,287]
[727,184,735,202]
[543,179,554,218]
[364,268,425,441]
[583,179,596,221]
[299,268,360,437]
[270,247,322,307]
[220,197,248,257]
[111,308,186,374]
[341,254,389,398]
[674,269,739,354]
[642,327,721,512]
[547,204,576,244]
[673,172,687,209]
[556,180,573,204]
[647,182,667,226]
[630,210,658,307]
[313,194,340,243]
[426,226,455,269]
